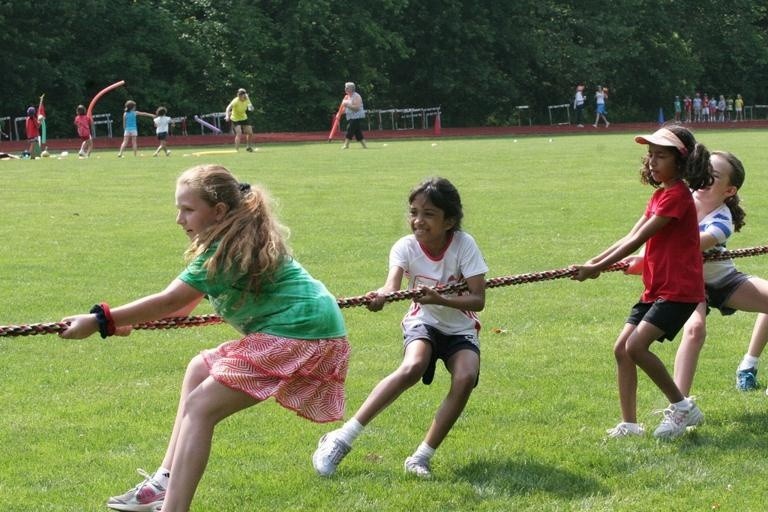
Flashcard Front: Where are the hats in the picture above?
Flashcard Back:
[28,107,36,115]
[634,129,687,160]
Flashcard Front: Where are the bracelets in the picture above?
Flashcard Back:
[99,303,117,337]
[90,304,110,338]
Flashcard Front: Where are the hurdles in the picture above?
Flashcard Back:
[90,113,114,139]
[0,115,28,143]
[335,106,442,131]
[547,103,571,127]
[168,111,234,137]
[744,103,768,120]
[516,104,533,127]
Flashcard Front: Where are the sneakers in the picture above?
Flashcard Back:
[403,457,432,480]
[604,423,644,438]
[654,398,704,439]
[736,367,759,392]
[312,431,351,476]
[106,471,166,512]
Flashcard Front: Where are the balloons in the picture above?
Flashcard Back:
[192,114,221,134]
[88,80,126,120]
[328,95,347,140]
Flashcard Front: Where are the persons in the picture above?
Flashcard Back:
[310,175,487,478]
[573,85,587,128]
[593,85,610,129]
[623,152,768,415]
[673,92,744,123]
[152,106,174,158]
[574,125,715,440]
[74,105,94,158]
[335,81,371,150]
[118,99,156,160]
[225,88,255,153]
[24,106,43,160]
[55,164,349,512]
